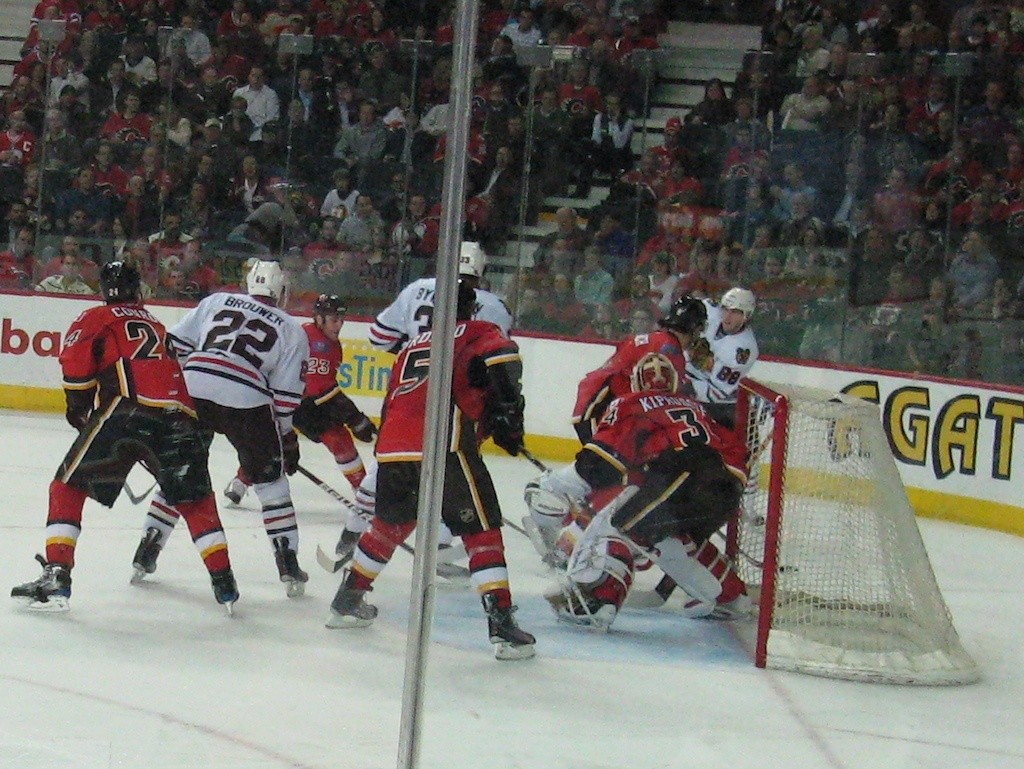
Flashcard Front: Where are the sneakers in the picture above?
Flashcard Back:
[273,535,308,598]
[223,476,248,508]
[481,593,537,660]
[324,569,378,629]
[710,589,751,620]
[211,569,239,615]
[560,598,616,634]
[334,527,363,558]
[130,527,163,584]
[10,554,72,613]
[435,542,471,588]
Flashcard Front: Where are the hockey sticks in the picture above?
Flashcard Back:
[123,481,158,506]
[715,529,799,572]
[624,429,774,608]
[371,428,532,539]
[316,431,490,574]
[500,516,529,537]
[295,465,469,565]
[517,442,598,519]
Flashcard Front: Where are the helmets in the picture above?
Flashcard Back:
[672,296,708,330]
[99,260,140,302]
[246,260,285,301]
[721,287,756,317]
[312,292,346,316]
[630,351,678,392]
[456,278,476,319]
[458,241,487,279]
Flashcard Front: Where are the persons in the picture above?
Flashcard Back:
[11,261,240,604]
[131,261,309,583]
[331,278,537,645]
[522,288,758,622]
[224,295,378,505]
[1,0,1024,386]
[336,241,513,555]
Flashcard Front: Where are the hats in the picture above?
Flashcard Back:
[205,118,221,129]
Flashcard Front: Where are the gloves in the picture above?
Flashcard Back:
[687,337,715,371]
[489,393,526,457]
[283,431,299,475]
[348,412,378,442]
[64,385,97,430]
